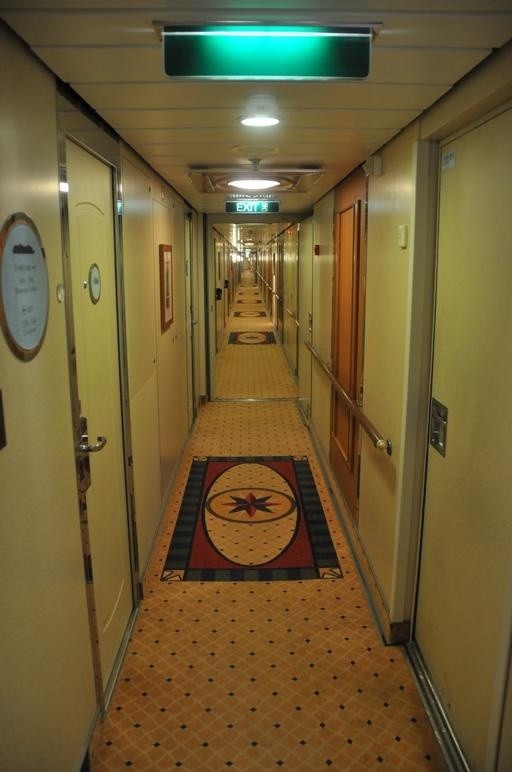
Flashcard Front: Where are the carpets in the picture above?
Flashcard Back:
[227,291,277,346]
[160,454,343,583]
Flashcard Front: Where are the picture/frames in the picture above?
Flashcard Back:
[158,242,175,331]
